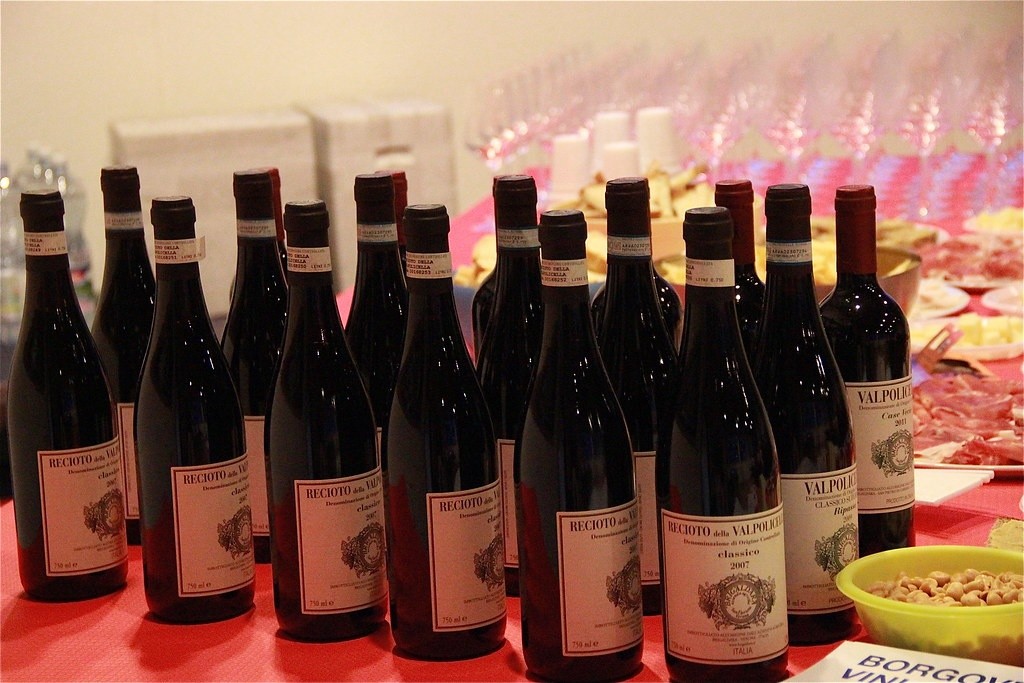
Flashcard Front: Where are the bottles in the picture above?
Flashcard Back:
[13,160,914,683]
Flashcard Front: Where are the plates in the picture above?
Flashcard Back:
[903,214,1023,363]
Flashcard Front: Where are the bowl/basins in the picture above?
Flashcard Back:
[836,545,1023,672]
[812,240,919,318]
